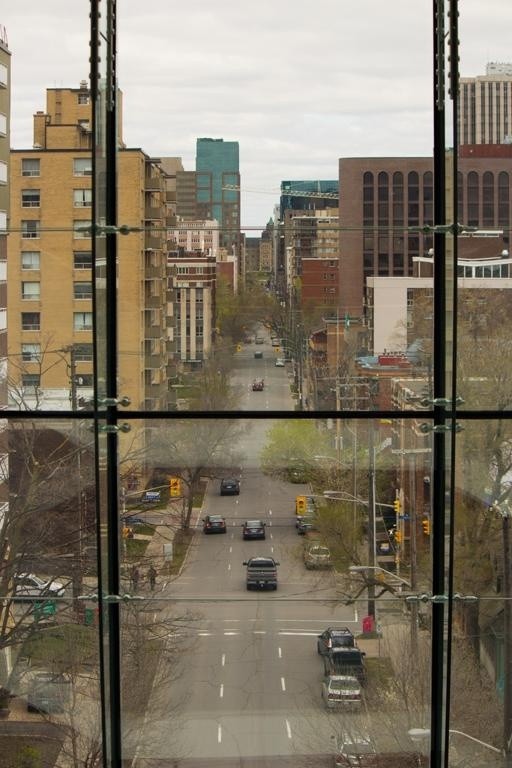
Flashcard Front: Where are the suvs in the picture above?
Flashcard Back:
[242,557,279,590]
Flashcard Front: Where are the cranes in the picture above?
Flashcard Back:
[222,176,339,200]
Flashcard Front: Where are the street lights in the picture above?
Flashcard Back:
[325,490,375,618]
[350,565,418,658]
[314,455,357,537]
[407,726,503,757]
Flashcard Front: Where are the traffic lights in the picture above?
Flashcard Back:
[170,479,181,498]
[395,531,402,543]
[420,520,430,534]
[395,501,400,513]
[123,528,133,539]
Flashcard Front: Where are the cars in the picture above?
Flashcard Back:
[15,572,68,603]
[288,458,307,482]
[28,671,68,710]
[276,358,284,366]
[255,337,263,344]
[255,351,262,358]
[201,515,226,534]
[305,544,330,570]
[295,497,319,534]
[220,478,240,496]
[241,520,266,539]
[270,333,279,346]
[317,625,365,711]
[252,378,263,391]
